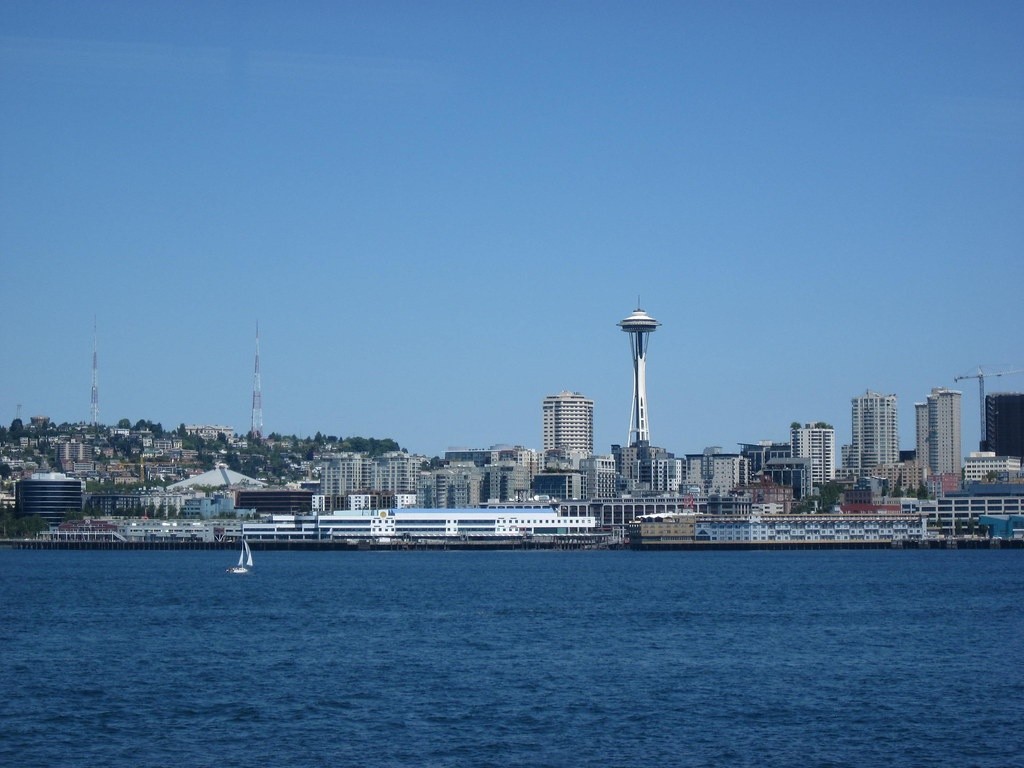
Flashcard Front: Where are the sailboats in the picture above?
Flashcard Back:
[225,535,254,573]
